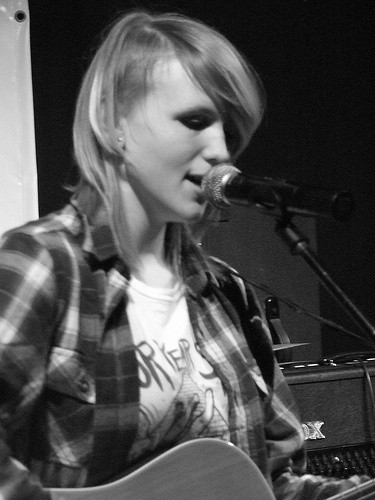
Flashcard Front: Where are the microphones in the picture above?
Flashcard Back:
[200,163,355,221]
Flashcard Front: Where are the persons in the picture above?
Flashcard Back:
[0,10,375,500]
